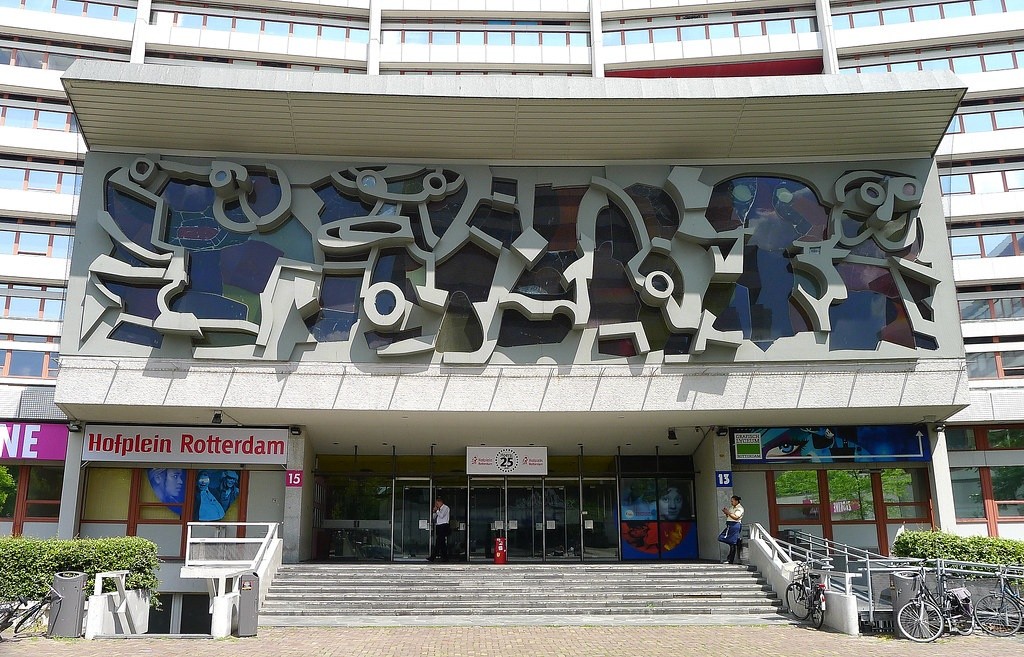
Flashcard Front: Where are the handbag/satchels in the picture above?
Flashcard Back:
[442,526,452,536]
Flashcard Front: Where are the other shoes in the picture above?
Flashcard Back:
[438,558,449,562]
[723,560,733,564]
[737,538,742,546]
[426,557,436,562]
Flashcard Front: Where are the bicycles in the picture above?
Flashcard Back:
[786,563,827,630]
[977,562,1024,639]
[898,558,976,643]
[0,580,63,642]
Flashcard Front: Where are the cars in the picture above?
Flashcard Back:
[358,535,402,560]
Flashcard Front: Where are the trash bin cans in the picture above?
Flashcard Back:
[784,529,802,560]
[238,569,261,639]
[48,571,88,639]
[893,570,924,639]
[494,536,507,564]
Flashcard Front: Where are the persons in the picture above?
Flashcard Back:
[148,468,183,513]
[717,496,745,564]
[213,470,240,512]
[658,479,685,520]
[195,471,225,521]
[663,522,683,550]
[426,499,450,562]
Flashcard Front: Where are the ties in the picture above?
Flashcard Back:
[436,508,440,518]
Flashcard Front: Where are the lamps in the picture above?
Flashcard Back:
[212,413,222,424]
[932,424,946,432]
[668,430,677,440]
[291,427,301,435]
[716,426,728,436]
[66,422,82,432]
